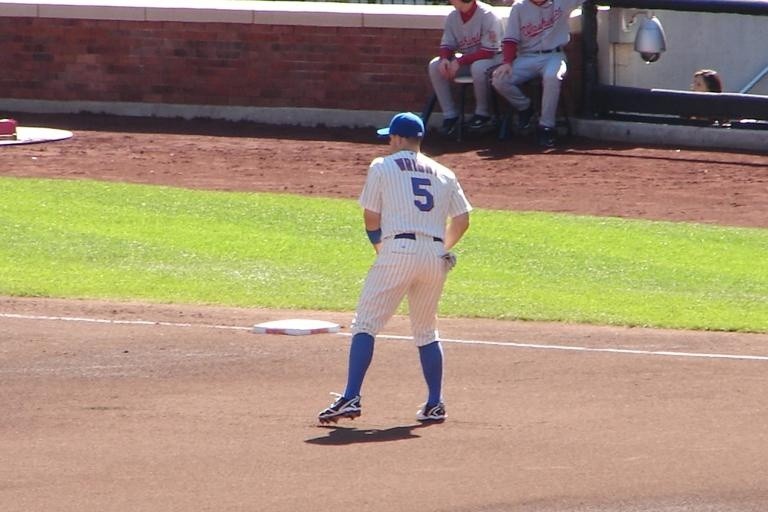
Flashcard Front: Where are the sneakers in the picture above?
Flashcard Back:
[541,125,557,148]
[317,392,364,423]
[466,113,493,128]
[517,104,536,128]
[414,401,448,422]
[439,116,460,138]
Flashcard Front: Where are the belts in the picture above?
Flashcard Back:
[533,45,563,56]
[394,231,444,244]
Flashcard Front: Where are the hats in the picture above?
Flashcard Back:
[374,110,428,138]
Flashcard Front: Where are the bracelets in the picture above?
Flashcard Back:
[366,228,382,244]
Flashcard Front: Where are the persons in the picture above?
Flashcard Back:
[686,69,729,122]
[317,111,472,427]
[427,0,505,138]
[491,0,587,154]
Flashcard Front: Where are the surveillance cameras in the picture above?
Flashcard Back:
[633,17,666,64]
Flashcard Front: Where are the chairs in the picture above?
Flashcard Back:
[421,75,498,140]
[498,77,572,139]
